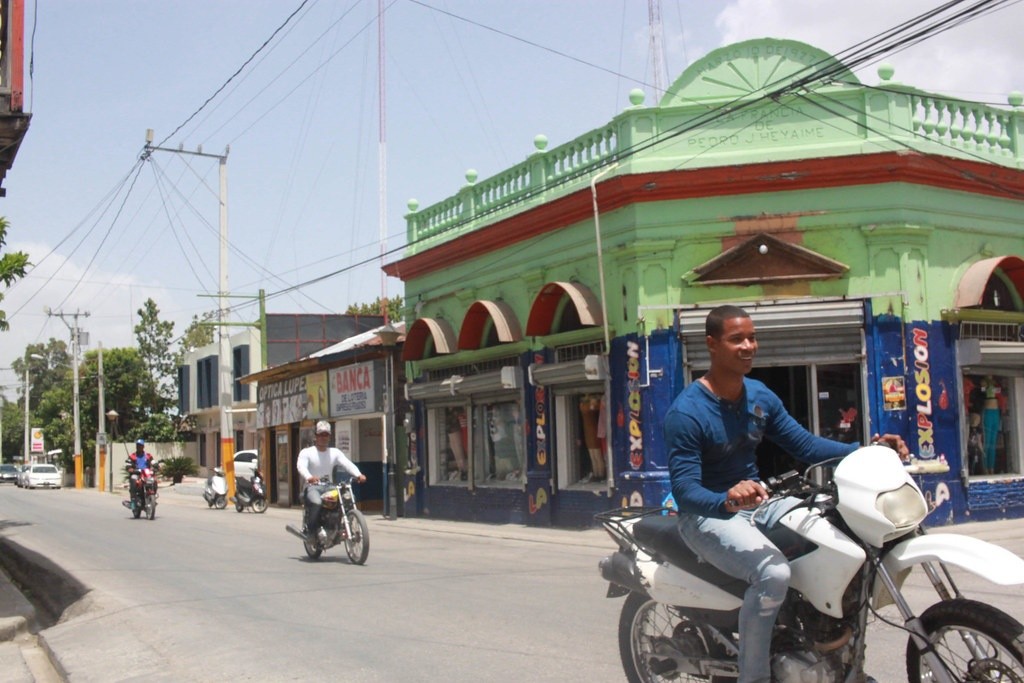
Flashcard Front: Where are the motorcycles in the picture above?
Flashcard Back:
[594,436,1024,683]
[120,459,165,520]
[286,476,370,565]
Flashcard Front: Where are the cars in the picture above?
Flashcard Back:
[0,465,20,484]
[232,449,258,480]
[17,464,62,489]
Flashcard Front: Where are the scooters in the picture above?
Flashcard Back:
[204,466,227,509]
[229,467,269,514]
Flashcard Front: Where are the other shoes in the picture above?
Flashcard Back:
[318,529,327,538]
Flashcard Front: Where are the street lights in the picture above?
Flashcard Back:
[31,354,85,488]
[106,409,120,493]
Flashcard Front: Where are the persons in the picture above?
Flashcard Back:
[665,305,909,683]
[297,420,366,529]
[964,383,1000,474]
[125,439,160,500]
[579,394,607,483]
[446,403,522,481]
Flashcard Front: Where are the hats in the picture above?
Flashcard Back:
[136,440,144,445]
[316,421,331,434]
